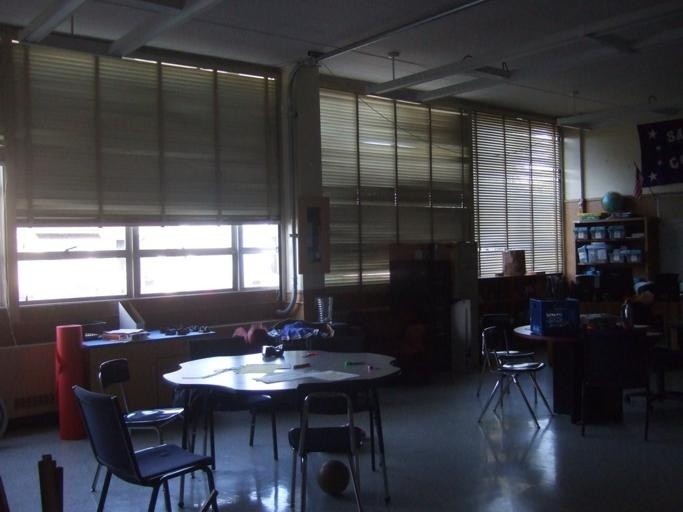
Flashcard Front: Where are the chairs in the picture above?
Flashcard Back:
[476,313,682,440]
[288,380,366,512]
[91,358,195,491]
[73,385,218,511]
[298,324,376,473]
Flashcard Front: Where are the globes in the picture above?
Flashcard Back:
[601,191,623,213]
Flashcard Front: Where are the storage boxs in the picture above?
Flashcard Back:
[502,250,525,275]
[530,298,580,336]
[574,225,643,265]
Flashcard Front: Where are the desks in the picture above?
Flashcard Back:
[161,350,400,504]
[81,329,216,409]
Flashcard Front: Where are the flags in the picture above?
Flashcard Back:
[631,165,643,200]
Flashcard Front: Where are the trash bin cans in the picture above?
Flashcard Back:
[297,322,368,412]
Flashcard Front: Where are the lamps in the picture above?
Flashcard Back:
[555,89,683,132]
[17,0,223,59]
[366,51,519,103]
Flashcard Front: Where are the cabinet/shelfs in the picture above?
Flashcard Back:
[574,216,660,314]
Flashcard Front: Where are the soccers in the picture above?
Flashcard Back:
[318,461,350,495]
